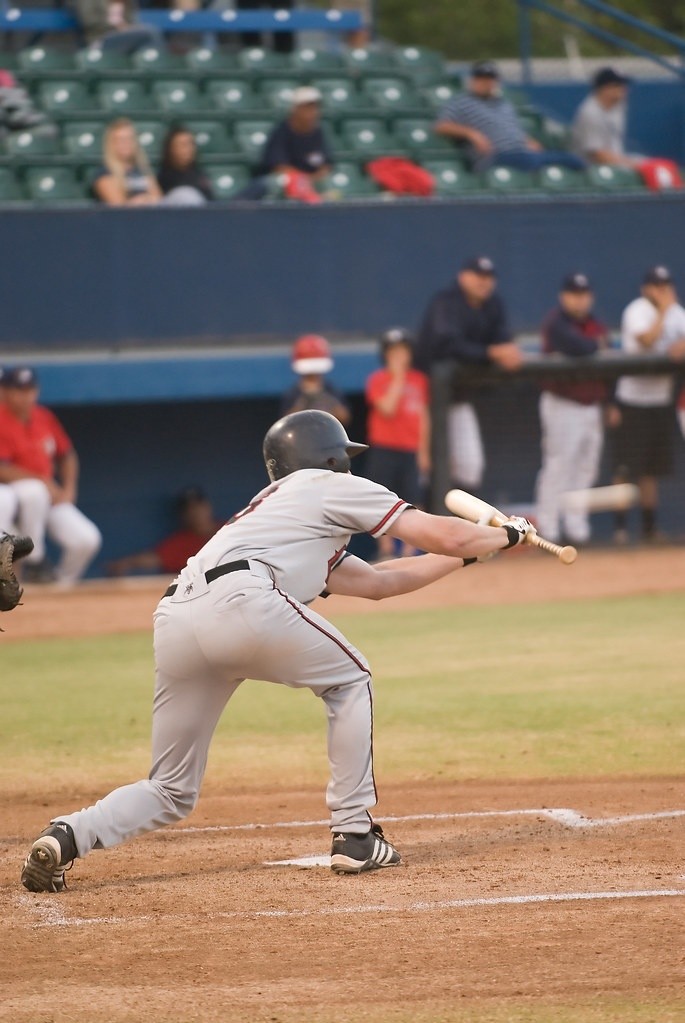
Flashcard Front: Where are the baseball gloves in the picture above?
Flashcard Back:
[0,531,35,612]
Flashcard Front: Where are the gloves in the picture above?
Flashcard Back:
[500,516,533,551]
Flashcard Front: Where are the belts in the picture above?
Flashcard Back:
[160,560,250,601]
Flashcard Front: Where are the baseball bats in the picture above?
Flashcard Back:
[444,489,578,566]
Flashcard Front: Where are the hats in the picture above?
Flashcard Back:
[643,267,671,283]
[594,68,629,85]
[462,254,494,274]
[471,60,499,78]
[3,368,36,389]
[291,87,323,106]
[560,270,595,293]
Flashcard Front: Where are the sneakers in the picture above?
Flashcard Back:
[330,823,402,874]
[21,821,77,893]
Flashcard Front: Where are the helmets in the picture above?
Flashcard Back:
[263,409,369,483]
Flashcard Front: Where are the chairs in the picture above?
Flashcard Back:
[1,34,645,205]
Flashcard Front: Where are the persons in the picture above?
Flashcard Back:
[85,86,341,204]
[434,57,587,171]
[609,264,685,542]
[0,364,101,586]
[285,335,349,426]
[364,325,432,556]
[568,64,648,172]
[115,485,227,572]
[535,274,612,543]
[21,409,538,893]
[414,255,517,494]
